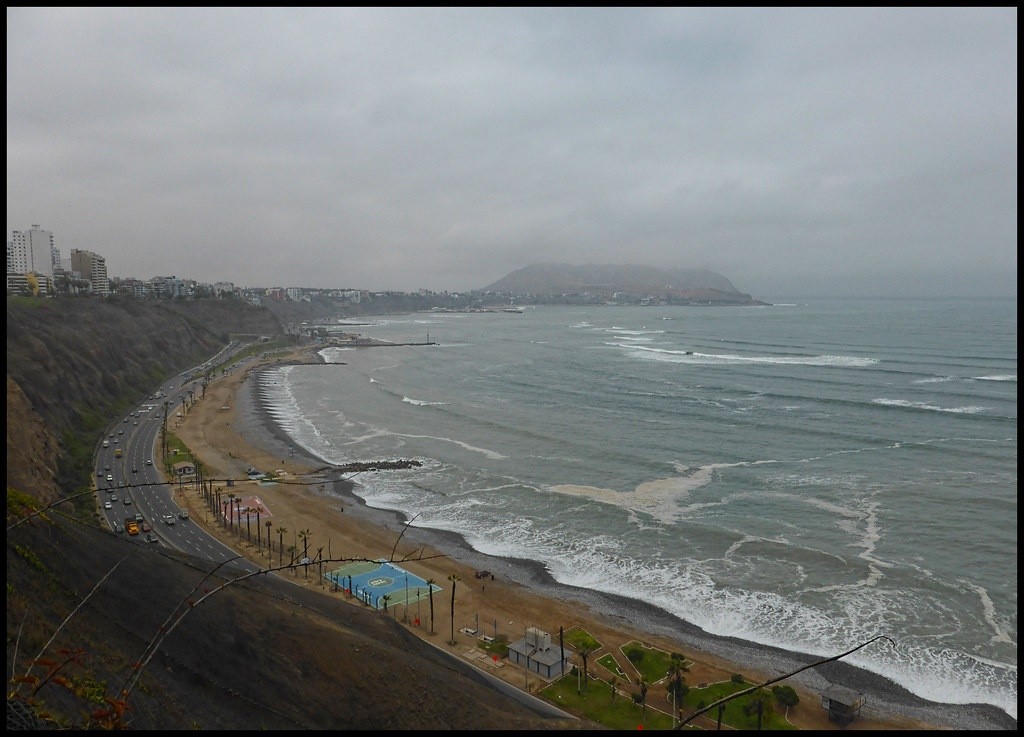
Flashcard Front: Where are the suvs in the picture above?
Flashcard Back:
[124,498,132,505]
[104,502,112,510]
[475,570,491,579]
[132,513,144,523]
[147,533,158,543]
[110,495,118,502]
[141,524,151,532]
[106,475,113,481]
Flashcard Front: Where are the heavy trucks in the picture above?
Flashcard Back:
[178,507,190,519]
[124,517,139,535]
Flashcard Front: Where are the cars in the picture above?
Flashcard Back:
[108,337,253,474]
[98,470,104,477]
[115,526,124,533]
[104,465,111,471]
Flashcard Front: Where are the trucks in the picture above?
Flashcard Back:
[166,515,176,525]
[103,441,109,449]
